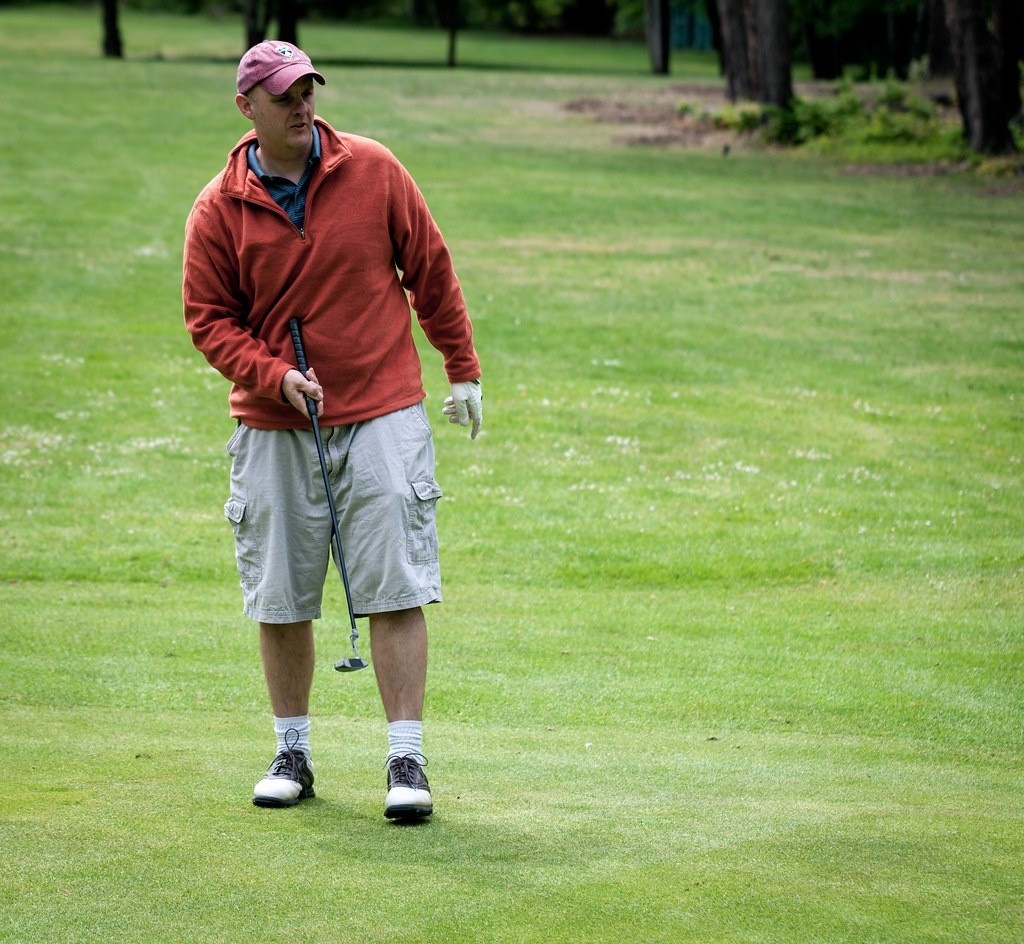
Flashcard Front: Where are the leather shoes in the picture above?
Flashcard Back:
[381,752,433,820]
[252,728,316,808]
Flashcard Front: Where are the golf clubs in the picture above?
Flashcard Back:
[288,317,370,673]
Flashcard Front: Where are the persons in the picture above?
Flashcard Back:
[181,40,483,818]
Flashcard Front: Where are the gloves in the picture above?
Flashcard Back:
[442,378,482,440]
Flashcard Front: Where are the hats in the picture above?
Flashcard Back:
[237,39,325,96]
